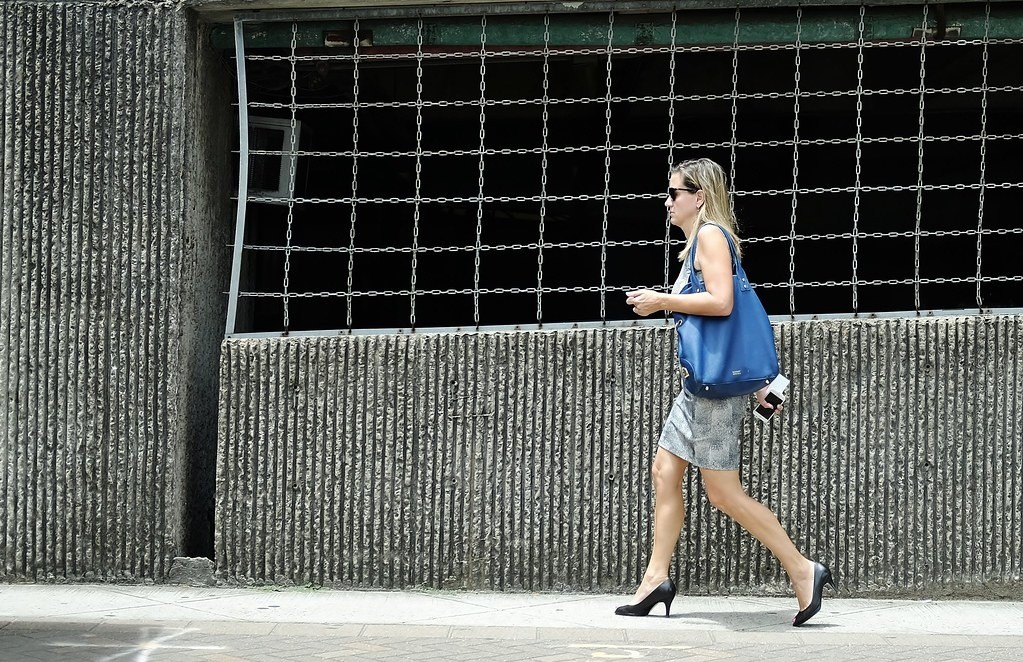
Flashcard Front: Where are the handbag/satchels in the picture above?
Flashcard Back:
[671,223,780,399]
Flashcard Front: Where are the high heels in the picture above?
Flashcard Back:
[792,562,841,626]
[615,578,676,617]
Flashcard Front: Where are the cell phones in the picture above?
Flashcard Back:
[753,389,786,423]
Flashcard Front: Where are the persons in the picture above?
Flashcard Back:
[615,159,839,628]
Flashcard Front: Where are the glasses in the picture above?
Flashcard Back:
[666,188,695,201]
